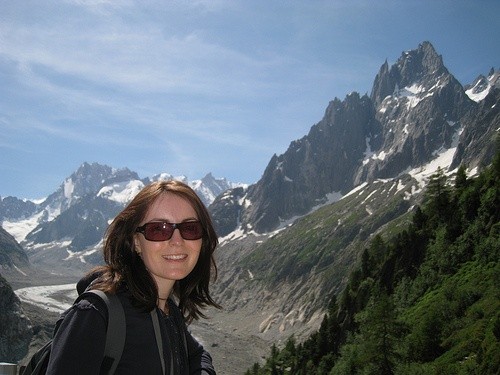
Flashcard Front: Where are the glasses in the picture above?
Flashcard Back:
[135,221,204,240]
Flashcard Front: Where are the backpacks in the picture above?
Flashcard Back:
[17,281,127,375]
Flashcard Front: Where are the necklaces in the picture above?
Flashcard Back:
[159,297,167,301]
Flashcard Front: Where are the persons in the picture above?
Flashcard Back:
[42,177,221,375]
